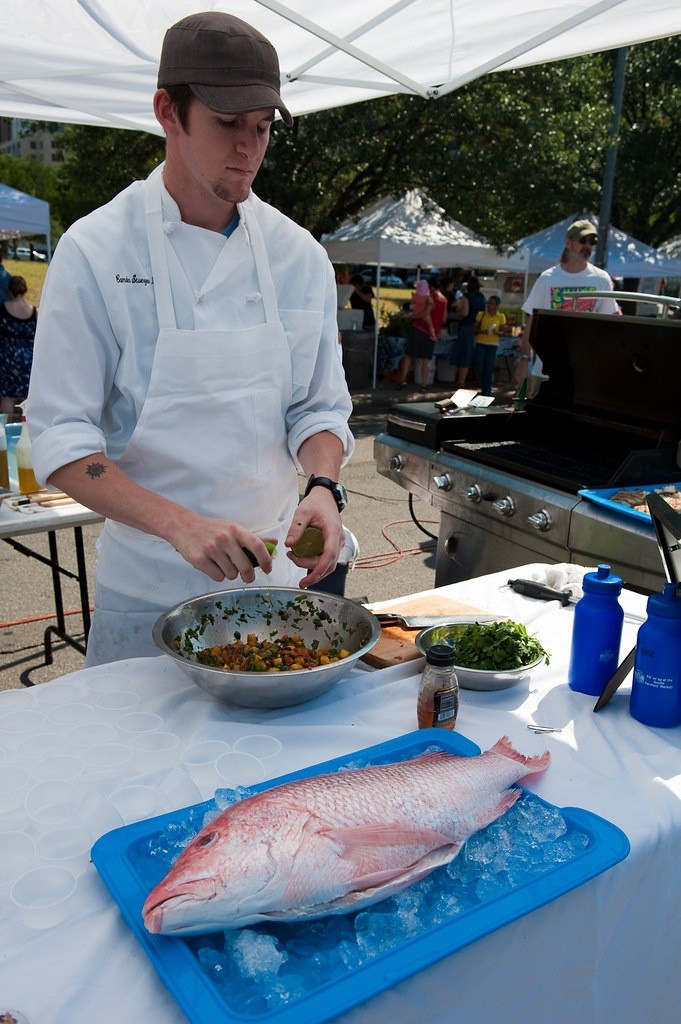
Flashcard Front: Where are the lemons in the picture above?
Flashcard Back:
[290,526,324,558]
[242,540,276,568]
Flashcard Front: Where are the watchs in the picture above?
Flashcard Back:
[304,474,349,513]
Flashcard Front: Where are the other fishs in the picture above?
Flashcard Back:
[142,738,552,933]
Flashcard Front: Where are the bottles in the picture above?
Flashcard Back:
[416,644,459,731]
[629,582,681,728]
[568,564,624,697]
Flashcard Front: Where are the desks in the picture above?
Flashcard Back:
[0,563,681,1021]
[0,493,108,663]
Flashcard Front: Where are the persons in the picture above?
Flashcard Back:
[27,11,360,670]
[0,256,38,424]
[513,219,623,385]
[333,263,507,396]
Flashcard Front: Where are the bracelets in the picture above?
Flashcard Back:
[520,354,530,361]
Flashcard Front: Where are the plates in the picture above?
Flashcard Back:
[576,483,681,526]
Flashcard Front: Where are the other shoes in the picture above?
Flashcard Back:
[394,381,408,390]
[421,386,429,392]
[450,383,464,388]
[430,337,439,342]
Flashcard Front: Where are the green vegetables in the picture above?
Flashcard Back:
[171,594,370,671]
[452,619,553,669]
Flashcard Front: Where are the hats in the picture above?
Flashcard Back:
[157,11,294,127]
[415,280,430,296]
[566,220,598,240]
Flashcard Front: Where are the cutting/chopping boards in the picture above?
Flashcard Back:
[358,595,491,668]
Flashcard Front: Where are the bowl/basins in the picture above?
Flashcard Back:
[152,586,382,709]
[415,622,544,691]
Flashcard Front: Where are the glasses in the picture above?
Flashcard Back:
[572,237,598,245]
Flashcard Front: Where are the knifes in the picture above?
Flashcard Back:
[373,614,509,631]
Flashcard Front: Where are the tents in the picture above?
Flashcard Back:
[0,183,51,266]
[512,208,681,324]
[0,1,681,138]
[320,184,530,389]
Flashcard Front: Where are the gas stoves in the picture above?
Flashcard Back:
[445,434,654,492]
